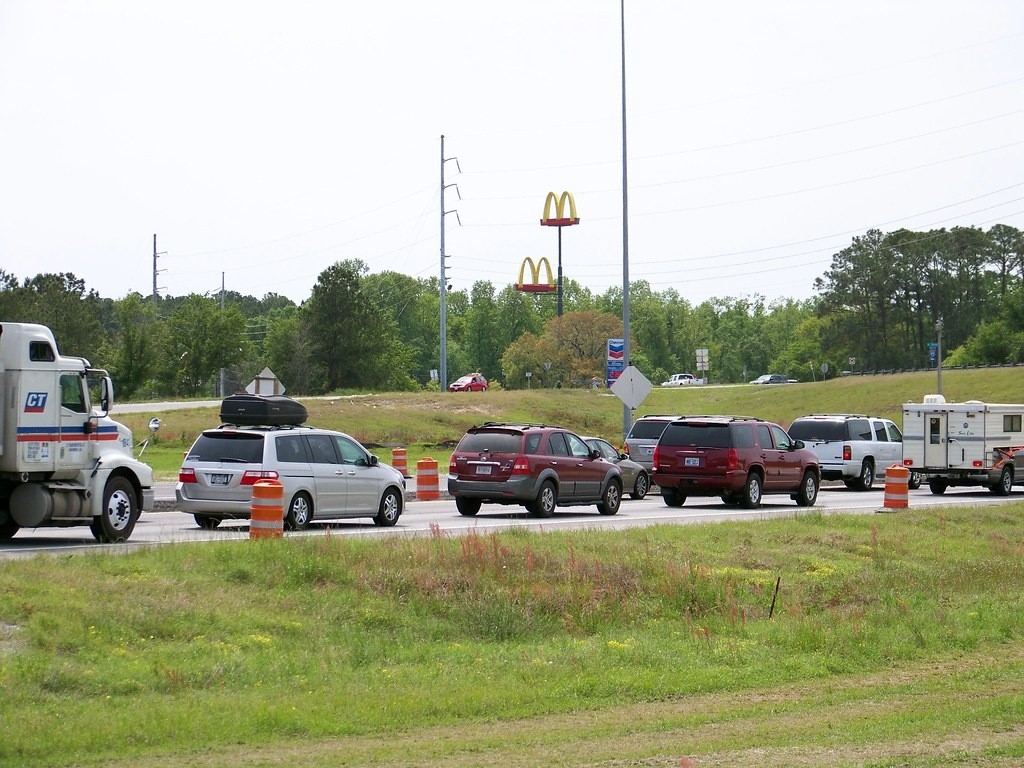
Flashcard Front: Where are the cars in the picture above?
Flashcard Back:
[449,372,487,393]
[569,434,650,500]
[747,374,789,384]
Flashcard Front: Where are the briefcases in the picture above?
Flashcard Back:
[219,394,309,425]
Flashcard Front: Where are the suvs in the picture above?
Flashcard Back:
[786,412,923,492]
[618,411,689,492]
[447,419,625,518]
[651,414,824,511]
[174,392,406,532]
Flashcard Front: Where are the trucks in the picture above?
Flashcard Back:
[0,320,156,547]
[900,392,1024,498]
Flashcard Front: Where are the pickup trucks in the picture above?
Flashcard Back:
[661,371,709,387]
